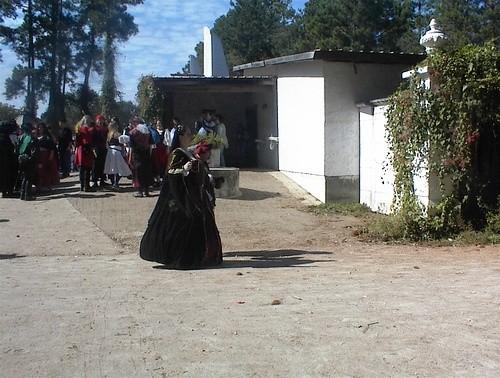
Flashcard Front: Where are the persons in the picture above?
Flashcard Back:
[0,111,228,202]
[139,124,223,268]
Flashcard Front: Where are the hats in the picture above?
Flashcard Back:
[96,115,104,120]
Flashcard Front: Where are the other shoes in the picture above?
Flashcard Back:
[0,173,162,201]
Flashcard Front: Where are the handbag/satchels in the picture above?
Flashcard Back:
[148,126,160,144]
